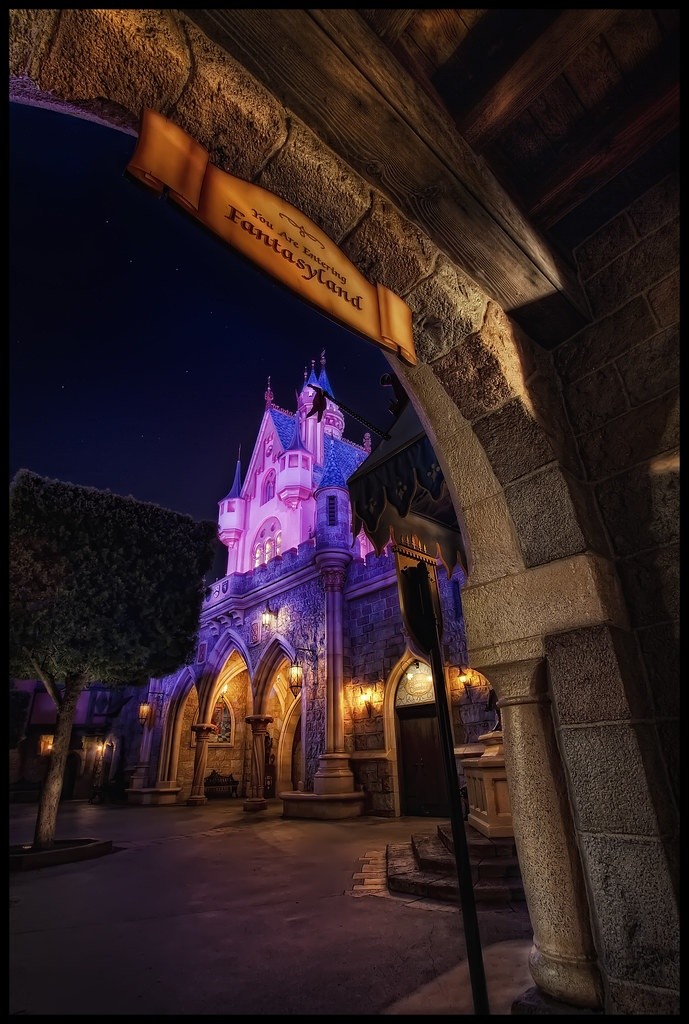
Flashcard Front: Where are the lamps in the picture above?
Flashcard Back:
[261,599,278,630]
[289,647,315,698]
[457,668,471,698]
[360,686,372,718]
[140,690,164,726]
[216,684,228,705]
[401,660,419,679]
